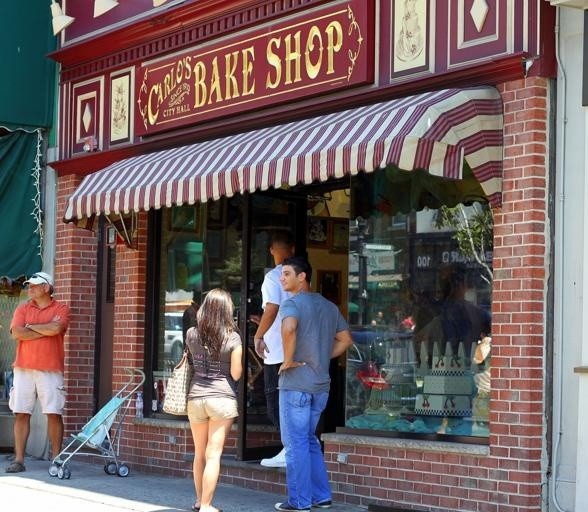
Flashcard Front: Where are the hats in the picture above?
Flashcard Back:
[23,272,54,286]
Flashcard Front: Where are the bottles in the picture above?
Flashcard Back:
[418,341,481,374]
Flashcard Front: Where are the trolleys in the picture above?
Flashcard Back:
[46,366,146,479]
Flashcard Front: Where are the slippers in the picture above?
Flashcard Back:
[192,504,199,511]
[5,462,26,472]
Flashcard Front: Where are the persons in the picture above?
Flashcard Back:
[5,271,72,475]
[413,262,492,436]
[178,286,245,512]
[272,253,355,512]
[251,232,312,469]
[180,286,211,351]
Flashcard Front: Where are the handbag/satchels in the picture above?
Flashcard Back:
[163,347,194,416]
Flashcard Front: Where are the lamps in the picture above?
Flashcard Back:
[49,0,76,38]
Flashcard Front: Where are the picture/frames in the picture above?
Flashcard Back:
[308,214,349,306]
[168,204,200,233]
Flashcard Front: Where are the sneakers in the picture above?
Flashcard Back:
[260,448,288,467]
[275,502,311,511]
[314,501,333,508]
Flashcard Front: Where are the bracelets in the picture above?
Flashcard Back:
[25,324,29,328]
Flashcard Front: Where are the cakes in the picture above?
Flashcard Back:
[470,371,490,396]
[414,394,471,418]
[469,396,489,420]
[383,343,408,365]
[469,421,490,438]
[376,363,413,384]
[429,354,466,377]
[365,384,423,413]
[423,376,473,394]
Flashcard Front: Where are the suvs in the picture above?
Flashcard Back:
[156,311,193,360]
